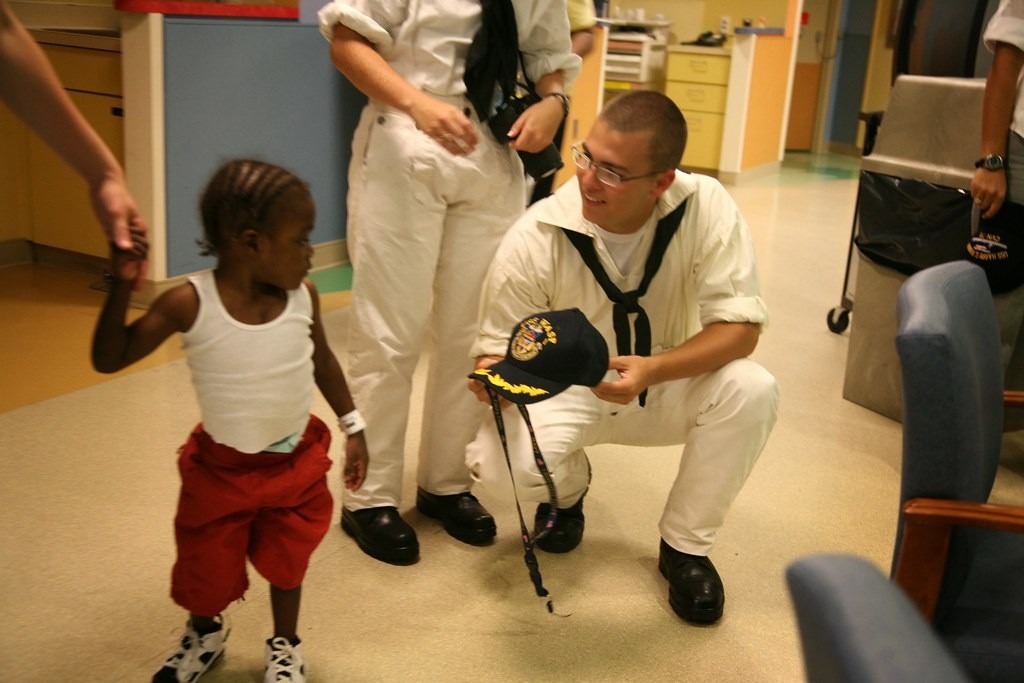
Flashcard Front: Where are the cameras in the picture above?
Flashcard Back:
[488,92,564,182]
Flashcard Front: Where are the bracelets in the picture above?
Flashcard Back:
[338,409,366,436]
[550,93,569,117]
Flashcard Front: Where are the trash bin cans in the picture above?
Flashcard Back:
[840,77,1024,422]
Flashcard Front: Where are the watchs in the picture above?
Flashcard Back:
[975,153,1003,171]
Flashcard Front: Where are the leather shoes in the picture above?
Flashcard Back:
[658,536,725,623]
[341,505,419,558]
[417,486,496,541]
[535,451,591,552]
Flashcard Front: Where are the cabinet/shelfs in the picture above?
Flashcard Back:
[0,24,124,267]
[595,18,732,173]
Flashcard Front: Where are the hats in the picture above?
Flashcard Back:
[468,308,609,405]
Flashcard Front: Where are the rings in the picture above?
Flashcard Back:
[442,133,451,140]
[975,197,982,205]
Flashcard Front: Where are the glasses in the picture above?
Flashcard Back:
[570,140,670,188]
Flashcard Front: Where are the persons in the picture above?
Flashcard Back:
[0,0,150,292]
[466,91,778,623]
[314,0,582,566]
[516,0,597,207]
[90,158,370,683]
[970,0,1024,379]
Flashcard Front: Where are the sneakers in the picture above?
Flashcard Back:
[264,636,305,683]
[153,612,226,683]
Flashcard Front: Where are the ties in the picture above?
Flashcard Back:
[561,199,687,406]
[462,1,519,124]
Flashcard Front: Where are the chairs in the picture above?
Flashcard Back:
[789,259,1024,682]
